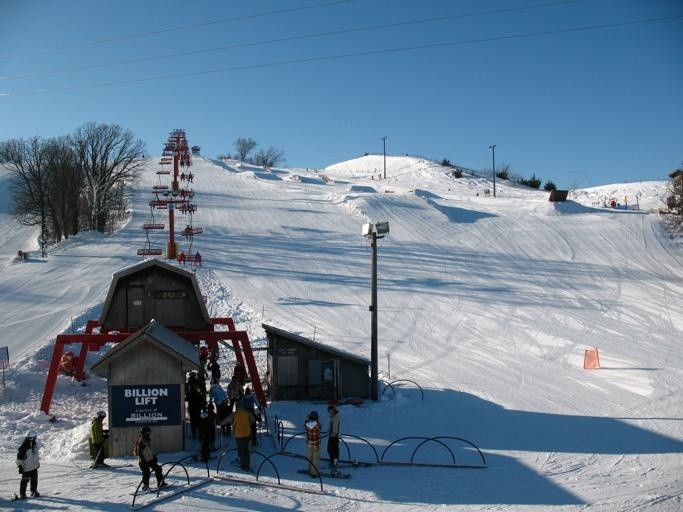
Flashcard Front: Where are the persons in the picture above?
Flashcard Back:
[15,430,41,500]
[89,411,109,468]
[133,426,168,491]
[179,251,186,265]
[181,172,193,184]
[181,189,194,199]
[180,159,191,168]
[182,203,194,214]
[185,225,194,238]
[326,405,342,477]
[193,251,202,266]
[184,345,262,471]
[302,411,322,479]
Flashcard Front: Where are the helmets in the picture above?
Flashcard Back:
[140,427,151,437]
[25,431,37,438]
[97,411,106,417]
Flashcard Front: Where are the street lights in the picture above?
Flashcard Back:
[381,136,387,179]
[360,221,389,400]
[488,144,496,196]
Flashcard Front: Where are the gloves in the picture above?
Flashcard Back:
[18,465,24,474]
[103,429,108,439]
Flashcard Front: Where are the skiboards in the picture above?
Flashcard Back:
[10,495,45,502]
[231,459,254,473]
[88,465,133,469]
[186,455,221,462]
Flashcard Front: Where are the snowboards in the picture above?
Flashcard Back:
[129,482,174,495]
[298,469,351,480]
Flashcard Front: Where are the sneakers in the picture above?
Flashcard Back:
[18,492,38,497]
[141,484,166,490]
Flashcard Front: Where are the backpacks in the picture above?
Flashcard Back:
[133,438,146,456]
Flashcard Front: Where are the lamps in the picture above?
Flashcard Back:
[377,221,389,235]
[362,222,374,237]
